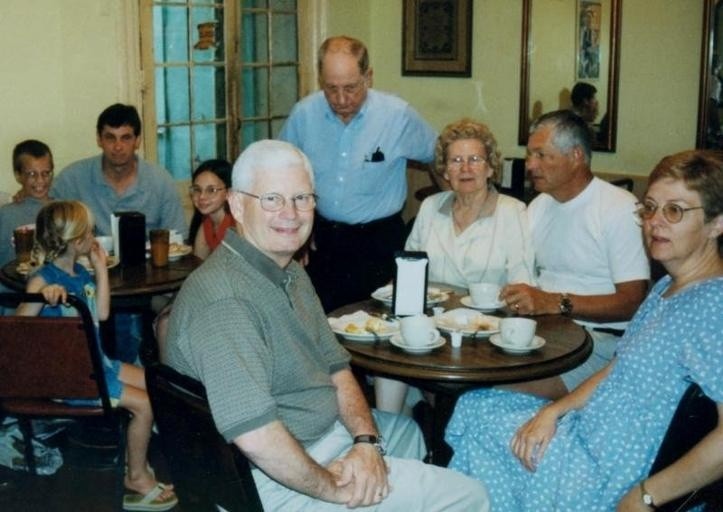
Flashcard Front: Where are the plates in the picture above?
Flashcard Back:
[324,282,546,357]
[146,244,193,263]
[73,254,120,275]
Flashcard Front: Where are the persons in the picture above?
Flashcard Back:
[582,6,599,79]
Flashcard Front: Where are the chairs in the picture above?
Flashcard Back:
[526,178,635,198]
[642,380,723,512]
[0,287,137,491]
[366,154,527,294]
[0,459,126,512]
[138,341,266,512]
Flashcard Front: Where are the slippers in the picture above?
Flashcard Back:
[114,449,179,511]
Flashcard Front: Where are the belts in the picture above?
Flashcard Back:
[313,212,402,235]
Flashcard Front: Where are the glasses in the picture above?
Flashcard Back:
[442,156,491,166]
[188,185,228,196]
[230,189,319,214]
[633,199,707,224]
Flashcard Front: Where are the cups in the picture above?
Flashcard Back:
[149,228,170,268]
[13,228,34,275]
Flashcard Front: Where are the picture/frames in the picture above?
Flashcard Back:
[516,0,622,153]
[693,1,723,156]
[400,0,472,80]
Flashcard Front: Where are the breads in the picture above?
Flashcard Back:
[468,321,489,332]
[168,243,192,256]
[345,316,388,335]
[76,255,116,268]
